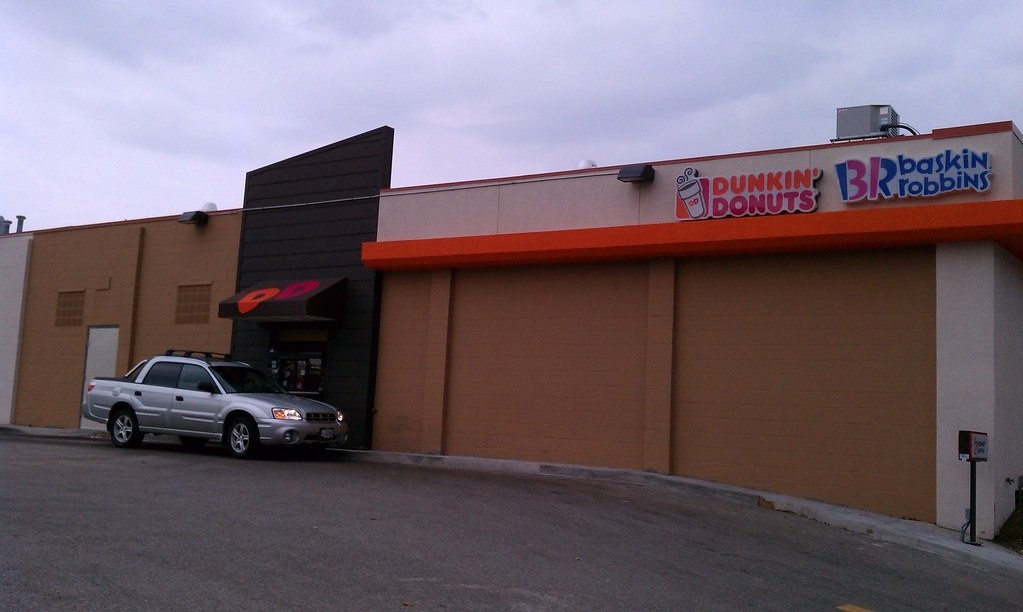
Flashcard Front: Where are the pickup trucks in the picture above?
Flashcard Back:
[80,348,349,461]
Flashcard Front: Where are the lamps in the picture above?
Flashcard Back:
[616,164,656,183]
[175,210,210,228]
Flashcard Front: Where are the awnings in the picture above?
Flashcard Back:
[217,277,349,323]
